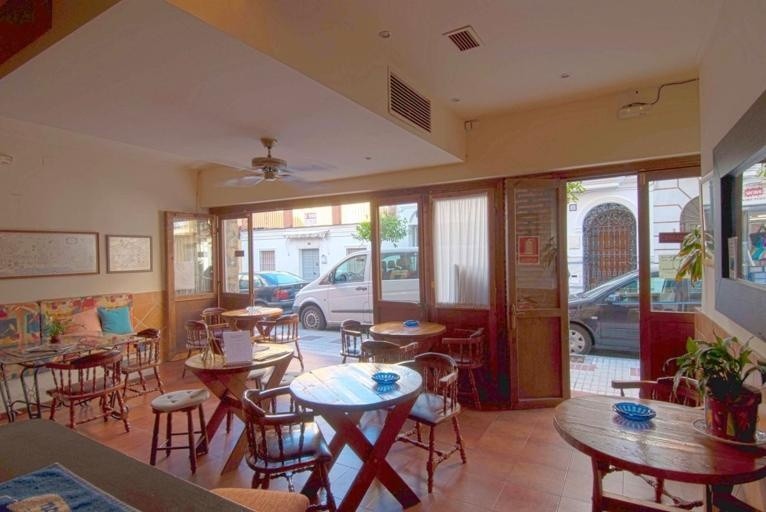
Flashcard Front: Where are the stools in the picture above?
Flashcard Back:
[149,386,213,476]
[210,485,311,512]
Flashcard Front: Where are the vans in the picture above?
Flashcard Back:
[292,246,419,331]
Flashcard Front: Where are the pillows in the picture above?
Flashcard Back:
[56,308,101,342]
[98,306,134,336]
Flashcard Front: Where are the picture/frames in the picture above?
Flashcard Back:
[0,231,101,277]
[105,234,153,274]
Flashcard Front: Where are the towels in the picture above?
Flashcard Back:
[0,460,144,512]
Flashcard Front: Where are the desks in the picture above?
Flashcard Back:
[1,417,259,512]
[552,395,765,512]
[291,362,422,512]
[185,342,296,475]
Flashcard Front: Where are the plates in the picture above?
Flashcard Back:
[611,403,657,421]
[371,371,401,384]
[691,418,766,447]
[403,319,419,327]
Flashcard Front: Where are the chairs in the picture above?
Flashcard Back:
[181,307,305,378]
[612,354,708,409]
[388,350,467,495]
[242,385,335,511]
[0,327,166,434]
[340,317,495,411]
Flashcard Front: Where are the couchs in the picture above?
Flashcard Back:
[0,292,161,414]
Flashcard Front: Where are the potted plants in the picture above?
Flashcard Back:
[661,325,765,443]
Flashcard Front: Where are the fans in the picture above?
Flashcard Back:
[219,134,316,191]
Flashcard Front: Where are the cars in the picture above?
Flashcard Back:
[239,270,310,313]
[567,268,702,355]
